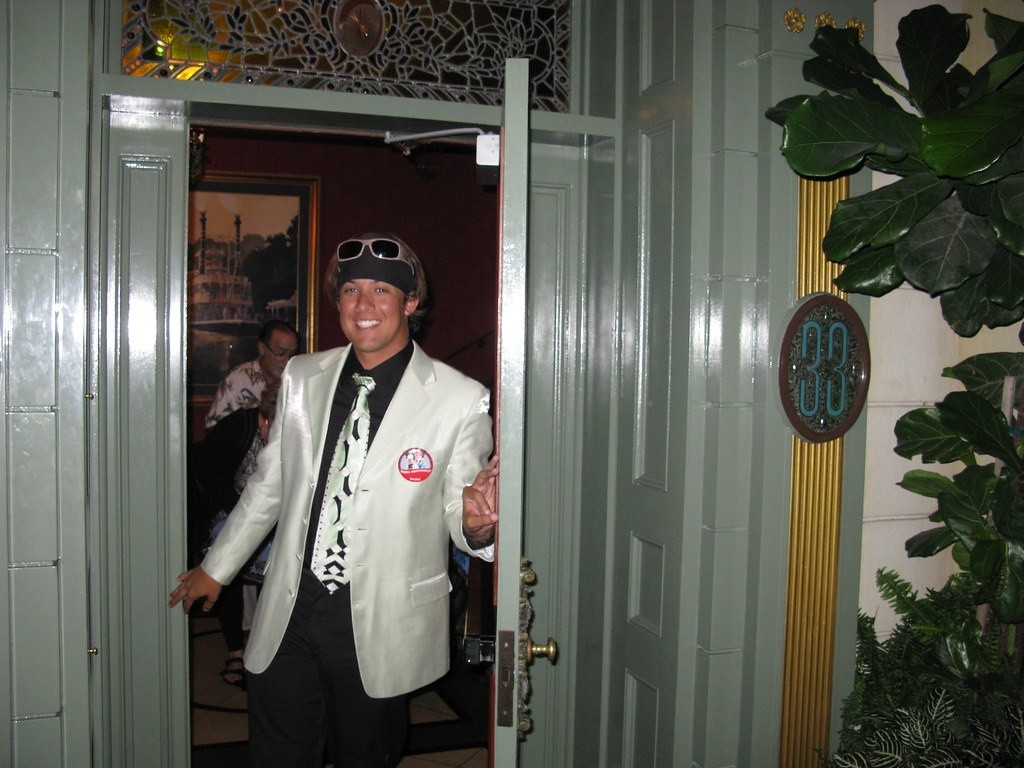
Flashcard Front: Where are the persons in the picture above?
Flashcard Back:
[203,323,298,686]
[169,234,499,768]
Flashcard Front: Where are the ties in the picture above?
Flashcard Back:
[310,373,377,595]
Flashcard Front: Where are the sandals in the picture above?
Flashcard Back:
[220,654,246,686]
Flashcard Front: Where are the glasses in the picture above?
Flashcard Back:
[261,339,297,362]
[335,238,415,278]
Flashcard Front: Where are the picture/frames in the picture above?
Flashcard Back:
[187,170,322,408]
